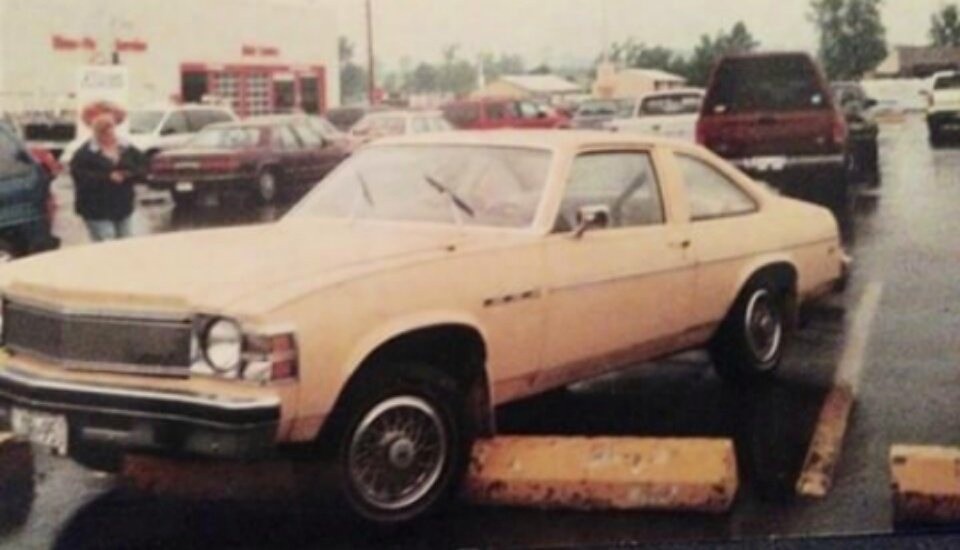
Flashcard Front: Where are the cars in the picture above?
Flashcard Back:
[828,78,878,160]
[346,82,710,143]
[151,111,356,204]
[0,109,57,243]
[58,100,240,187]
[916,68,960,135]
[0,128,852,541]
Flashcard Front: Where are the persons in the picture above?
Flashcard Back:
[70,116,149,241]
[47,101,147,245]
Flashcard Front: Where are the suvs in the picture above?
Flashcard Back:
[691,51,850,178]
